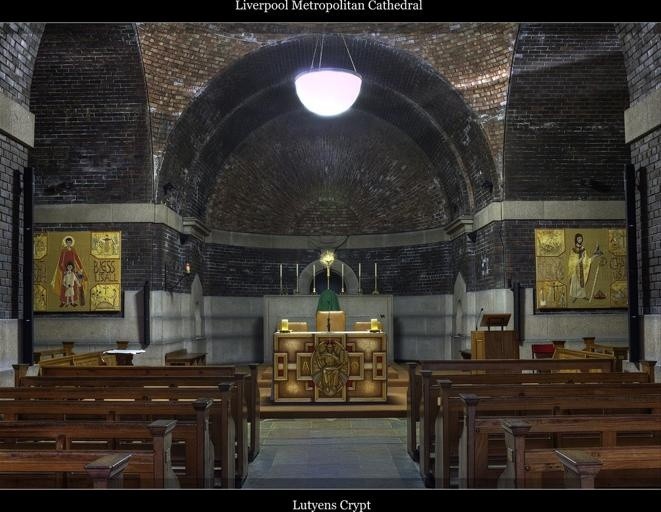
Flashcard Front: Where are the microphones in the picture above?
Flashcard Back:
[327,312,330,331]
[476,308,483,331]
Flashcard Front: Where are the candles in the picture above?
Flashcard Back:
[278,262,381,279]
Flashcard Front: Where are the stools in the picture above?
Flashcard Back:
[461,348,473,358]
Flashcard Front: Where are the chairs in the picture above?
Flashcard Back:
[531,343,557,375]
[316,311,347,331]
[277,321,310,333]
[355,322,383,332]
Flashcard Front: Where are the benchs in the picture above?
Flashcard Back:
[0,362,263,489]
[164,348,208,365]
[403,358,661,489]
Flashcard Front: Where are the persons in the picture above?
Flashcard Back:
[63,262,84,308]
[314,339,350,395]
[567,233,601,304]
[51,236,91,309]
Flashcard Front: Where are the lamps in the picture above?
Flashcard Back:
[321,247,335,266]
[292,21,364,118]
